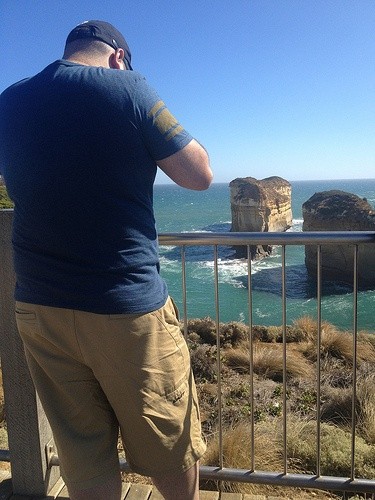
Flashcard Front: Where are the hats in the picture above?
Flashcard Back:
[65,20,134,73]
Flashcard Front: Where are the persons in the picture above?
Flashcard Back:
[0,20,214,500]
